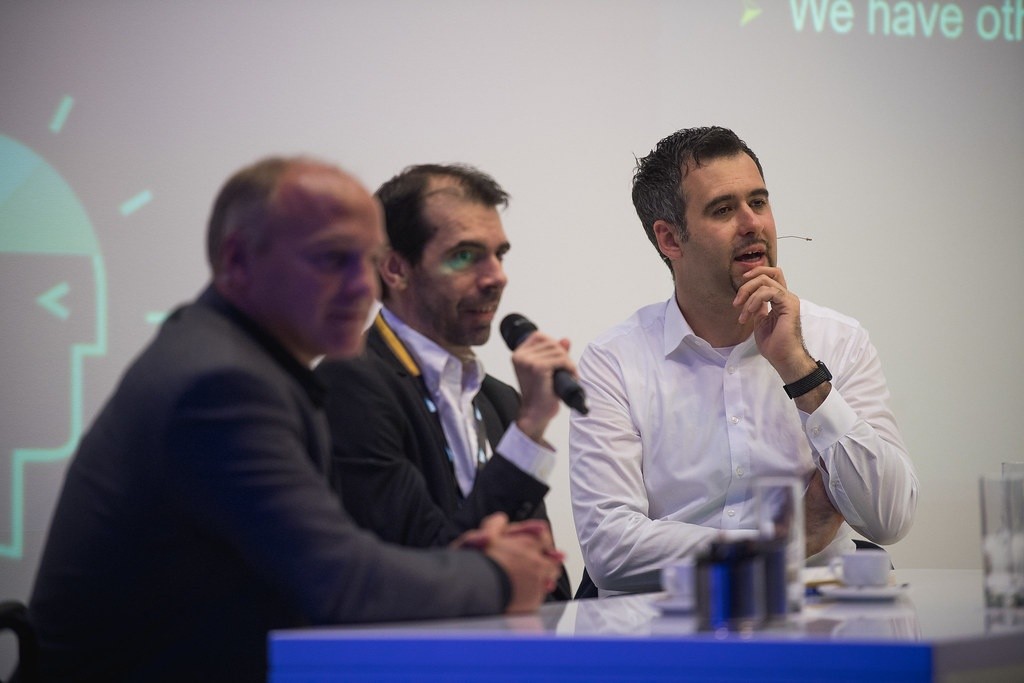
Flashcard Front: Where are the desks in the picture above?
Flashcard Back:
[266,568,1024,683]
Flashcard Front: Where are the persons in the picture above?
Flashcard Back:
[565,126,916,598]
[310,163,572,602]
[30,156,565,683]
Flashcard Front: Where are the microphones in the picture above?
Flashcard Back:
[500,314,588,414]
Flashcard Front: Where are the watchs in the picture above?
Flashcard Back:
[784,360,832,397]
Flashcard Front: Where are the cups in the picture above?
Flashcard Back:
[830,550,891,589]
[662,563,694,601]
[979,473,1024,609]
[754,475,805,614]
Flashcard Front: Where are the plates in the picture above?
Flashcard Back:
[649,598,696,613]
[817,584,903,600]
